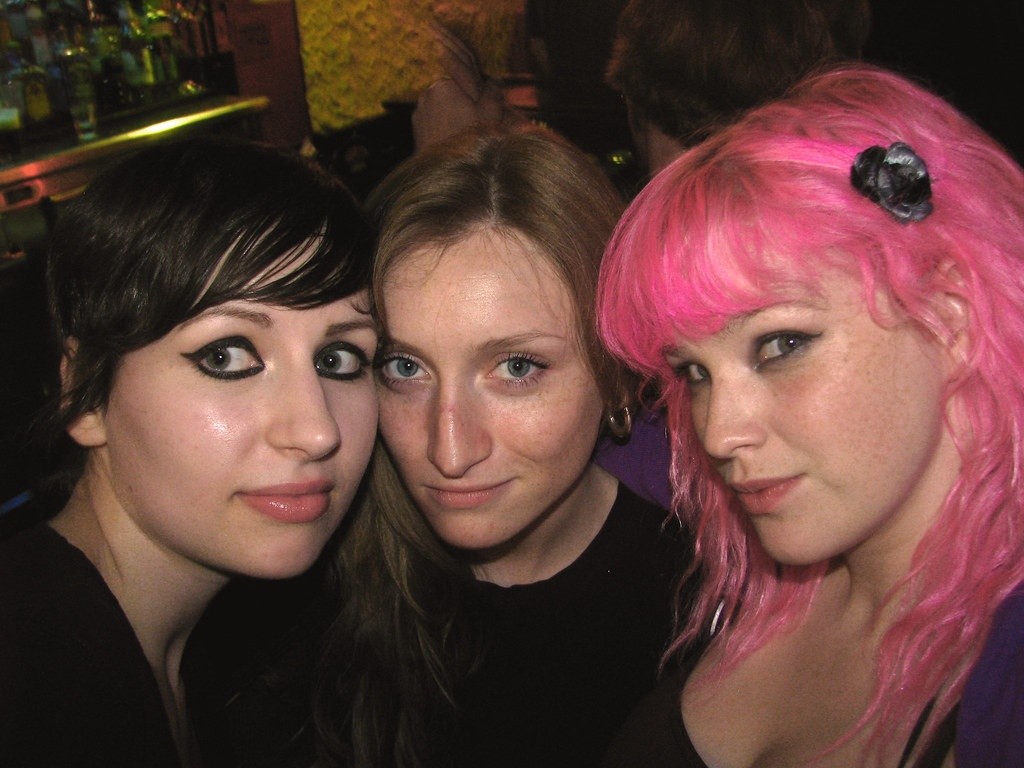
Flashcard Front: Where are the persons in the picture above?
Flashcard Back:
[310,121,728,768]
[592,60,1024,768]
[0,131,384,768]
[294,0,869,522]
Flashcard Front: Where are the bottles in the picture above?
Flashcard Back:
[0,0,236,162]
